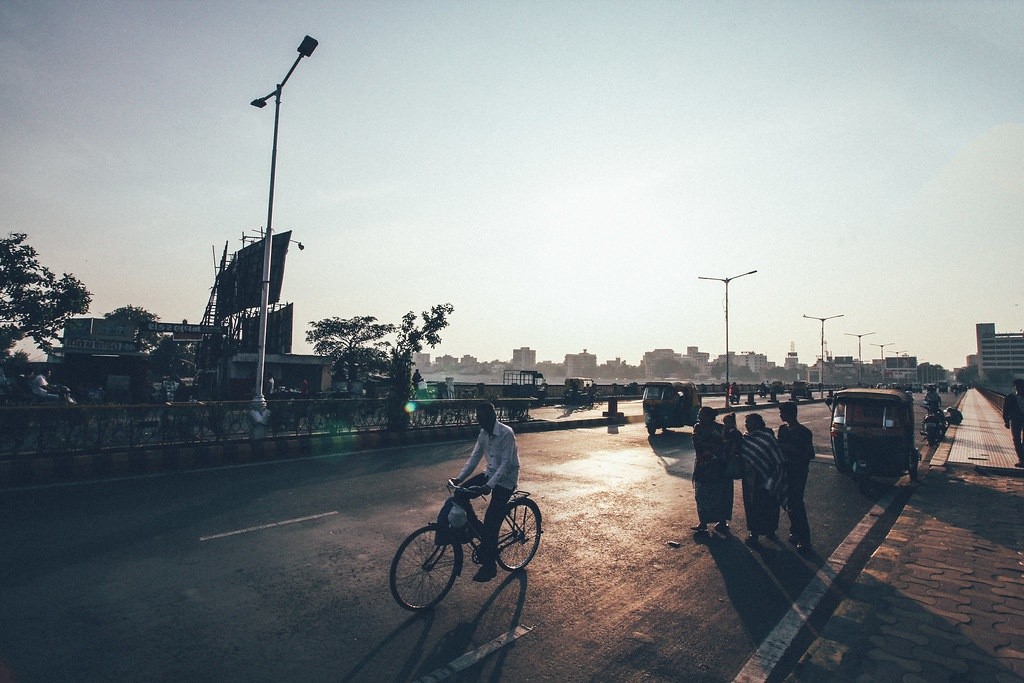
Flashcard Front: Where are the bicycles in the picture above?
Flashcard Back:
[388,477,544,613]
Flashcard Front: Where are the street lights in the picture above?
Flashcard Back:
[803,313,844,398]
[250,33,319,450]
[845,331,910,389]
[697,269,758,405]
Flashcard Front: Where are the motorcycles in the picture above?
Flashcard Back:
[640,379,702,437]
[920,406,945,444]
[757,388,767,398]
[825,388,920,495]
[27,385,86,426]
[730,393,740,404]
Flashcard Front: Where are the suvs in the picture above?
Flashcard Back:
[790,381,813,399]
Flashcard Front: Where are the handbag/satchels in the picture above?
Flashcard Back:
[434,497,477,545]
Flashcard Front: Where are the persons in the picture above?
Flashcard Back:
[777,402,815,553]
[446,403,521,583]
[737,412,781,549]
[690,406,734,532]
[1002,379,1024,468]
[720,412,743,467]
[7,357,968,425]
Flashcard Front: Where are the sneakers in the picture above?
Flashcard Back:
[473,563,498,583]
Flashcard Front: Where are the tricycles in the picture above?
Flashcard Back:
[771,380,785,394]
[502,369,603,410]
[878,380,949,397]
